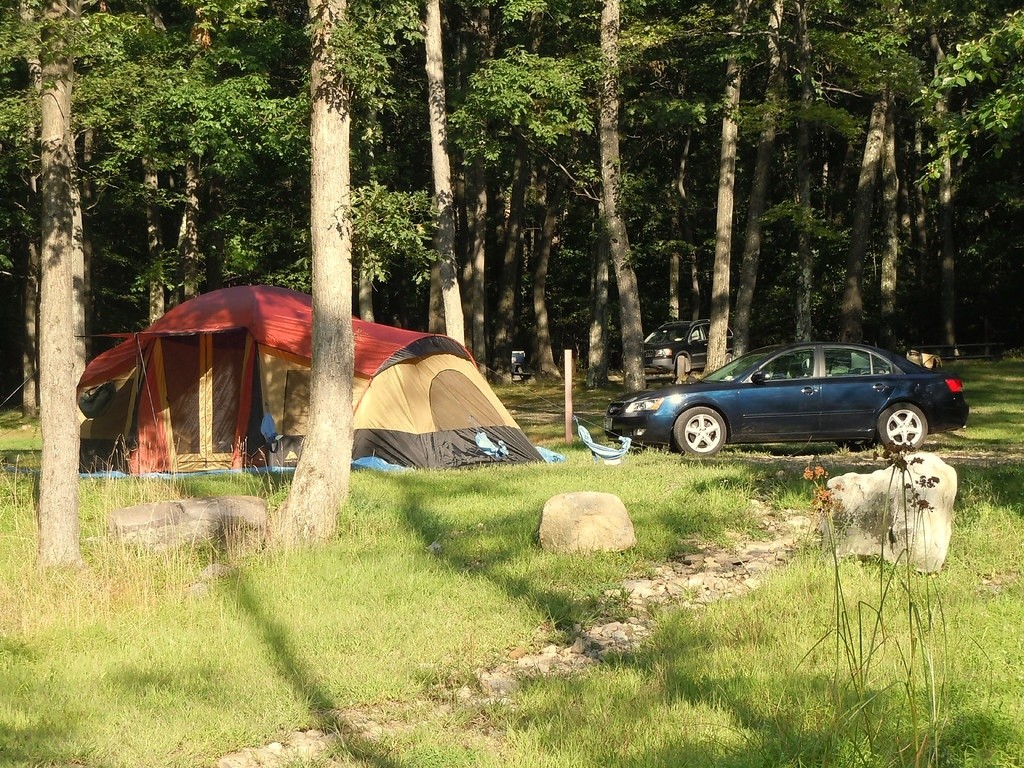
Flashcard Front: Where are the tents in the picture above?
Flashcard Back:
[76,286,548,478]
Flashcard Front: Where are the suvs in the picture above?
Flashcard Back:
[643,319,734,380]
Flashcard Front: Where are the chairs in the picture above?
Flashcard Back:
[241,414,284,471]
[802,358,811,378]
[511,351,535,382]
[692,329,699,340]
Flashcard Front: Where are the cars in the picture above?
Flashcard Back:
[604,340,970,457]
